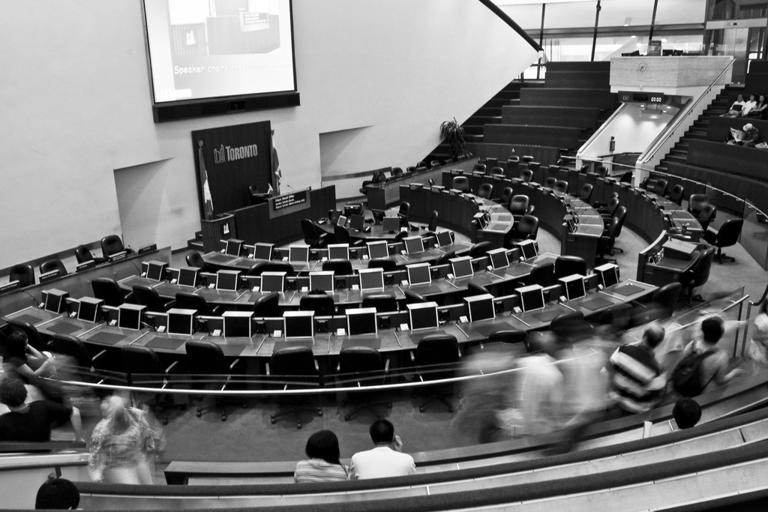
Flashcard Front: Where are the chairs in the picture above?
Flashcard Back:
[360,151,473,193]
[598,303,634,323]
[674,246,717,307]
[186,341,249,421]
[476,329,530,352]
[551,311,585,329]
[338,345,392,421]
[452,155,743,263]
[7,319,186,423]
[635,282,682,317]
[408,334,462,413]
[622,50,686,55]
[265,347,323,429]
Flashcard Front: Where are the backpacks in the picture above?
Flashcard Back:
[672,340,720,398]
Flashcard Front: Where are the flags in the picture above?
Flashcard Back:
[199,147,213,220]
[271,135,282,194]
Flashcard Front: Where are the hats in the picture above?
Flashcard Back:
[743,123,752,131]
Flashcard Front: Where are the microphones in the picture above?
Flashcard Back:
[287,184,299,191]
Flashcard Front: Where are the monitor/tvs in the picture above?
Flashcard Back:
[433,231,454,249]
[406,301,440,333]
[371,209,390,225]
[40,269,61,282]
[43,288,69,314]
[485,248,511,271]
[146,261,169,281]
[177,267,202,288]
[405,262,433,286]
[514,285,547,313]
[402,235,425,256]
[166,308,198,336]
[253,242,276,262]
[308,270,335,294]
[462,294,497,324]
[473,213,488,228]
[288,245,312,264]
[138,244,158,256]
[215,269,241,292]
[225,239,246,257]
[260,271,288,294]
[107,250,128,264]
[77,295,105,324]
[515,242,538,262]
[327,243,350,261]
[0,279,21,293]
[558,272,587,303]
[222,309,255,340]
[365,240,390,261]
[76,259,95,272]
[349,213,363,233]
[382,216,400,235]
[283,310,316,340]
[344,307,379,340]
[117,303,148,331]
[593,263,621,288]
[449,255,474,280]
[357,267,386,294]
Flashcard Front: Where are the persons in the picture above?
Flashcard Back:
[0,322,167,485]
[449,285,767,457]
[35,478,80,510]
[347,419,417,481]
[293,429,350,484]
[720,93,768,148]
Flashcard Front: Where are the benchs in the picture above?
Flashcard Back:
[483,123,588,150]
[748,58,768,73]
[745,72,768,89]
[542,69,610,87]
[727,89,768,119]
[518,87,619,107]
[706,116,768,148]
[500,103,601,125]
[686,139,768,182]
